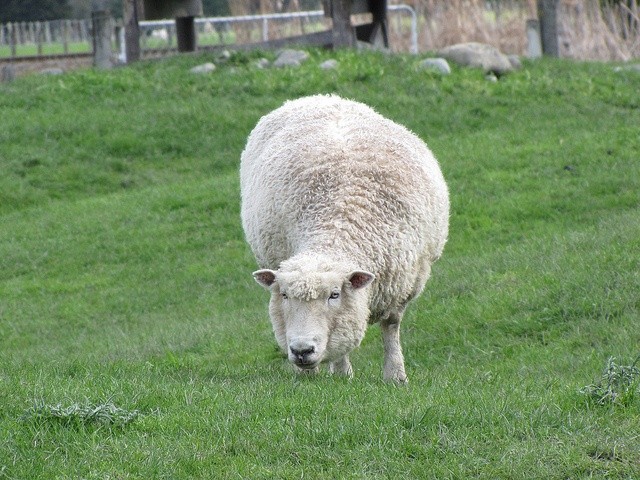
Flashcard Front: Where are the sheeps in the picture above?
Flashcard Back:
[239,92,452,390]
